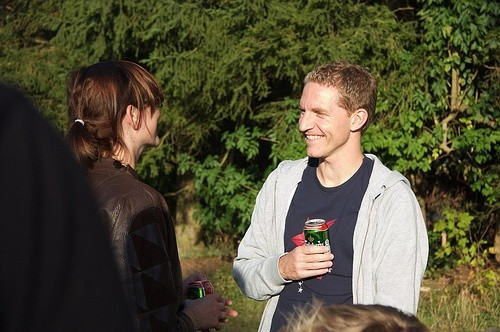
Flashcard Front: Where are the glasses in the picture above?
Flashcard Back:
[138,97,162,110]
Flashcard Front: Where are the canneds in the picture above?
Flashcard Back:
[185,280,214,300]
[303,218,331,262]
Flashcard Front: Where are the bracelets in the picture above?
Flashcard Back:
[183,306,200,330]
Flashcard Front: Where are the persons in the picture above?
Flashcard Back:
[232,62,433,332]
[63,59,239,332]
[0,79,137,332]
